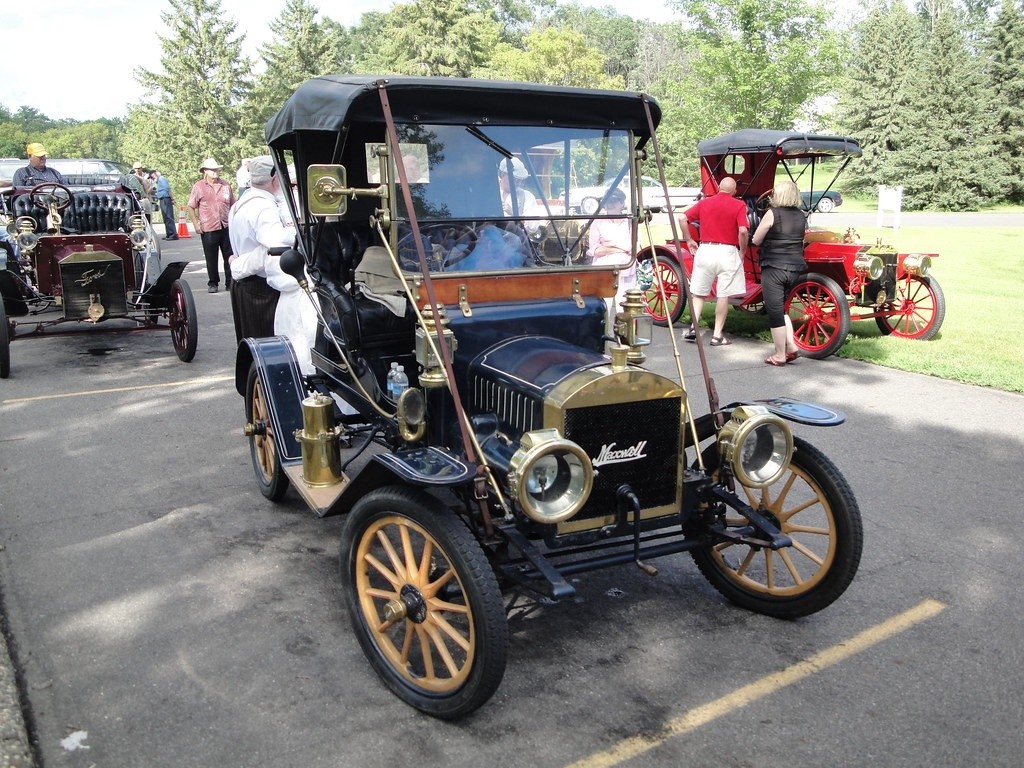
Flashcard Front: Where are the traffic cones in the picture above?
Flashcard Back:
[177,205,192,238]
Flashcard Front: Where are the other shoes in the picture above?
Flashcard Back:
[225,283,231,291]
[209,284,218,293]
[161,234,179,240]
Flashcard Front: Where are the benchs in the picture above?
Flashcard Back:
[13,189,133,235]
[309,219,539,404]
[732,193,774,283]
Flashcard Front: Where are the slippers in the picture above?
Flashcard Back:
[786,350,801,362]
[764,356,785,366]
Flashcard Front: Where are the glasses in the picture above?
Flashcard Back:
[606,198,622,203]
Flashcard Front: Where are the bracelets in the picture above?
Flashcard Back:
[171,200,174,201]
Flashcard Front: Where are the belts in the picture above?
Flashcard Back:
[700,241,736,246]
[160,197,171,200]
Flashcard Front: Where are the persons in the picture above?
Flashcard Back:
[235,158,251,199]
[499,156,541,234]
[228,188,322,376]
[128,161,153,226]
[287,163,298,190]
[187,158,237,293]
[12,143,67,186]
[752,180,807,366]
[147,170,179,240]
[586,187,641,313]
[676,177,751,347]
[227,155,297,346]
[403,155,428,183]
[0,228,44,297]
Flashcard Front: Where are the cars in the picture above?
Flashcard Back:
[232,72,866,723]
[801,190,842,214]
[0,172,199,379]
[559,174,703,216]
[632,128,947,360]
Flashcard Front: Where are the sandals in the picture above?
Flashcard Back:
[684,329,697,341]
[710,336,733,346]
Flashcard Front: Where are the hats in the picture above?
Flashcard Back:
[26,143,49,157]
[249,154,279,176]
[148,170,156,179]
[605,188,626,203]
[499,156,528,181]
[199,158,224,173]
[133,162,142,169]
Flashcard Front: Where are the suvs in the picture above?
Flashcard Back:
[0,158,160,226]
